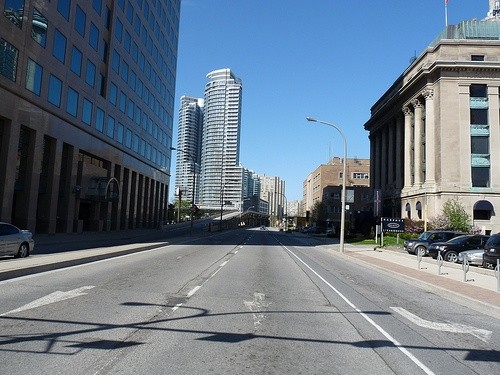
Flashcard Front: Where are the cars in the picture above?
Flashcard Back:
[458,248,485,266]
[0,221,35,258]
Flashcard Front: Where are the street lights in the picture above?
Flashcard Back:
[170,146,196,236]
[221,179,238,222]
[305,116,348,253]
[274,192,287,233]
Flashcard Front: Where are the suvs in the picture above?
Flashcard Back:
[403,231,462,257]
[260,225,265,230]
[483,232,500,267]
[428,234,489,264]
